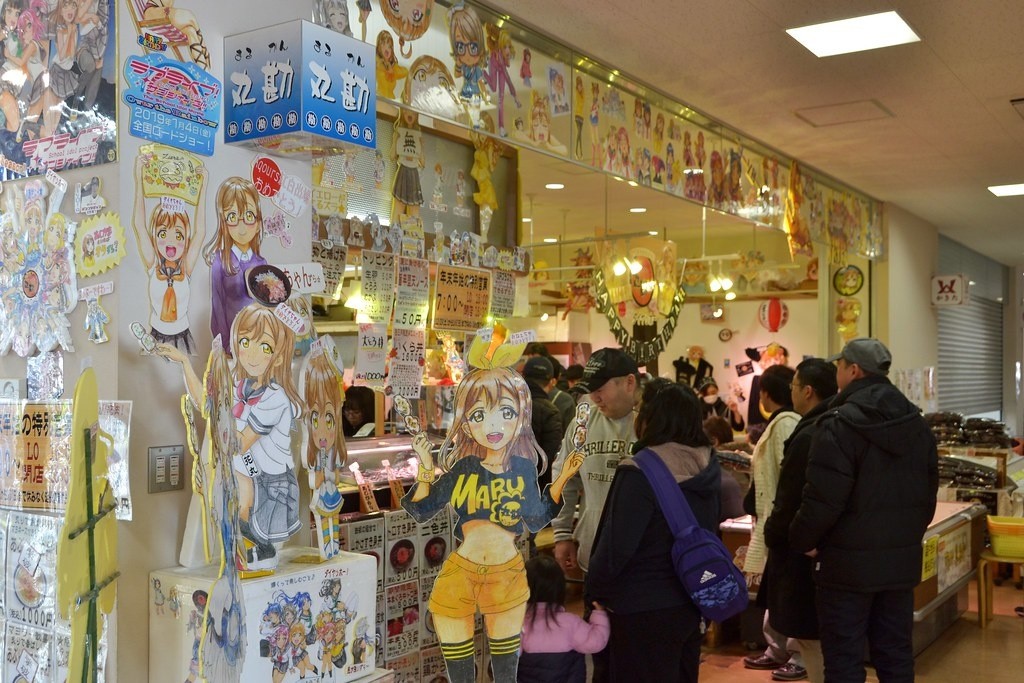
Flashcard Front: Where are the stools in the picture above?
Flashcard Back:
[976,548,1024,629]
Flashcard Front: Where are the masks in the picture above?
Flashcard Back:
[703,394,718,405]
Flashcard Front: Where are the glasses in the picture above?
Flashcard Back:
[789,383,804,390]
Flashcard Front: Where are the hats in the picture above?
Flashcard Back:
[824,337,892,375]
[522,356,554,379]
[567,347,637,394]
[697,377,717,392]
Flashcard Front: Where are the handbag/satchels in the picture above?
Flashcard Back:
[632,447,750,623]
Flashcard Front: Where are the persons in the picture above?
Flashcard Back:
[339,385,385,514]
[522,342,585,529]
[551,348,768,683]
[742,338,938,683]
[517,556,611,683]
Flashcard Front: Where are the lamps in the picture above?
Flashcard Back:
[716,273,733,291]
[624,254,642,274]
[344,280,361,309]
[710,304,722,318]
[706,274,721,292]
[723,288,736,301]
[610,255,626,276]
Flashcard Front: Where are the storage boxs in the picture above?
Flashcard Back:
[148,544,378,683]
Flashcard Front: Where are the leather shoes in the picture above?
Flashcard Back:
[770,662,808,681]
[743,653,783,670]
